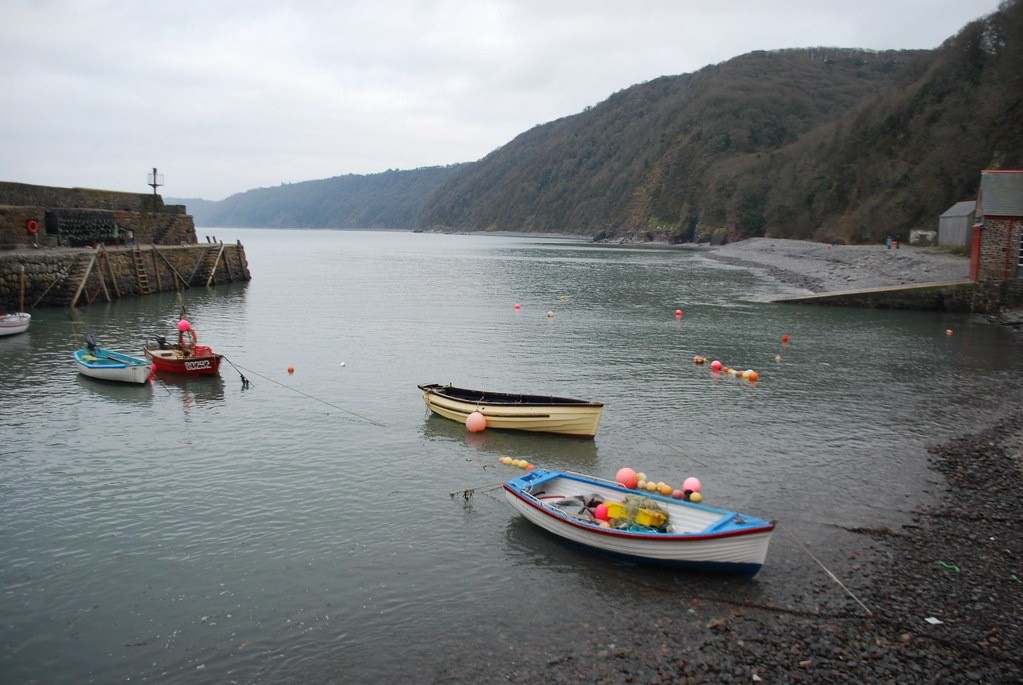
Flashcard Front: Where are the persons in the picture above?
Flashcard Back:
[886,235,892,248]
[896,234,900,249]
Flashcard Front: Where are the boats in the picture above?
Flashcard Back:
[0,312,31,335]
[142,305,222,378]
[418,382,604,441]
[73,333,153,387]
[500,468,777,580]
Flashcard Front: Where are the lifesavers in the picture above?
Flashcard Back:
[178,327,197,350]
[27,220,38,233]
